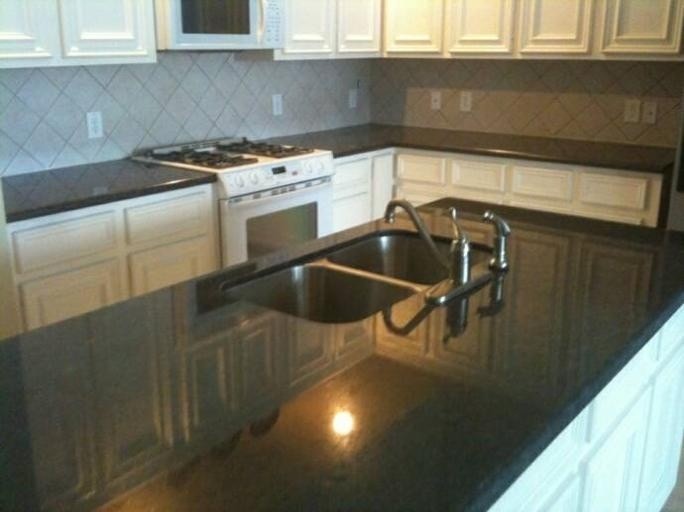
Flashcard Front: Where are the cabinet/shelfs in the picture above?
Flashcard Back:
[271,0,384,64]
[392,147,668,227]
[333,147,392,230]
[8,174,221,338]
[384,1,520,62]
[521,1,684,63]
[0,0,160,68]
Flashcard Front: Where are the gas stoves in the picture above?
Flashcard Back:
[131,136,335,194]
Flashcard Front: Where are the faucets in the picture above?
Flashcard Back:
[383,199,471,286]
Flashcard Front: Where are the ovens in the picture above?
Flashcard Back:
[218,178,336,267]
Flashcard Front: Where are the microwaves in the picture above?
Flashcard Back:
[156,1,283,54]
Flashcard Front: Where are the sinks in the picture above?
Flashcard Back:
[327,229,494,286]
[218,263,423,324]
